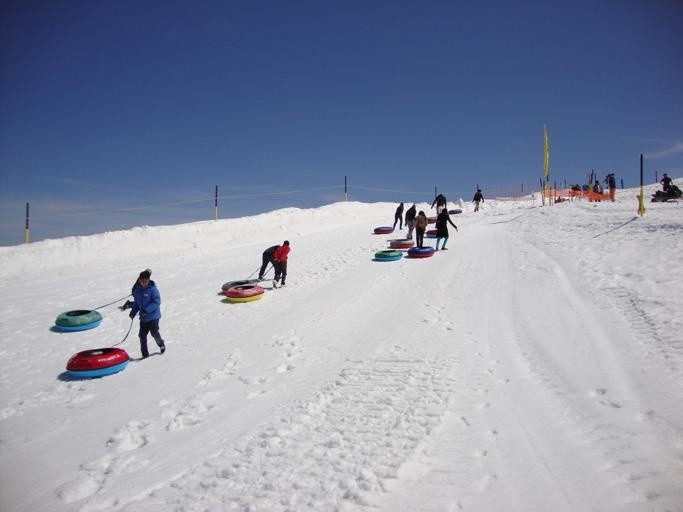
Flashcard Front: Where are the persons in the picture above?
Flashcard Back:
[272,240,290,289]
[123,268,152,310]
[405,204,416,240]
[660,174,673,202]
[393,203,403,230]
[472,189,484,212]
[258,245,282,281]
[413,211,428,248]
[435,208,458,251]
[129,271,165,360]
[431,194,446,228]
[608,174,616,202]
[592,181,603,202]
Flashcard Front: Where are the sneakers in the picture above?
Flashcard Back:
[160,340,165,353]
[272,279,277,288]
[441,247,447,250]
[281,284,287,287]
